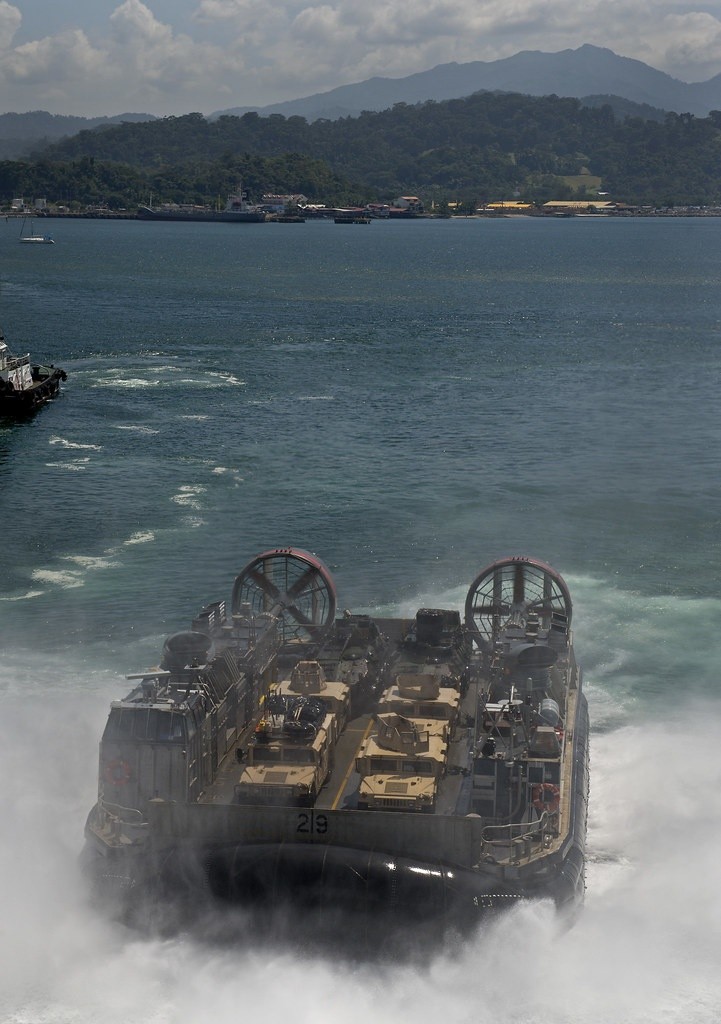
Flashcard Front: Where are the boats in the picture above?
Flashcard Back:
[0,336,67,420]
[135,205,268,222]
[75,546,590,917]
[271,215,305,223]
[335,216,372,224]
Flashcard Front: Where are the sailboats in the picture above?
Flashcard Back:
[18,197,55,244]
